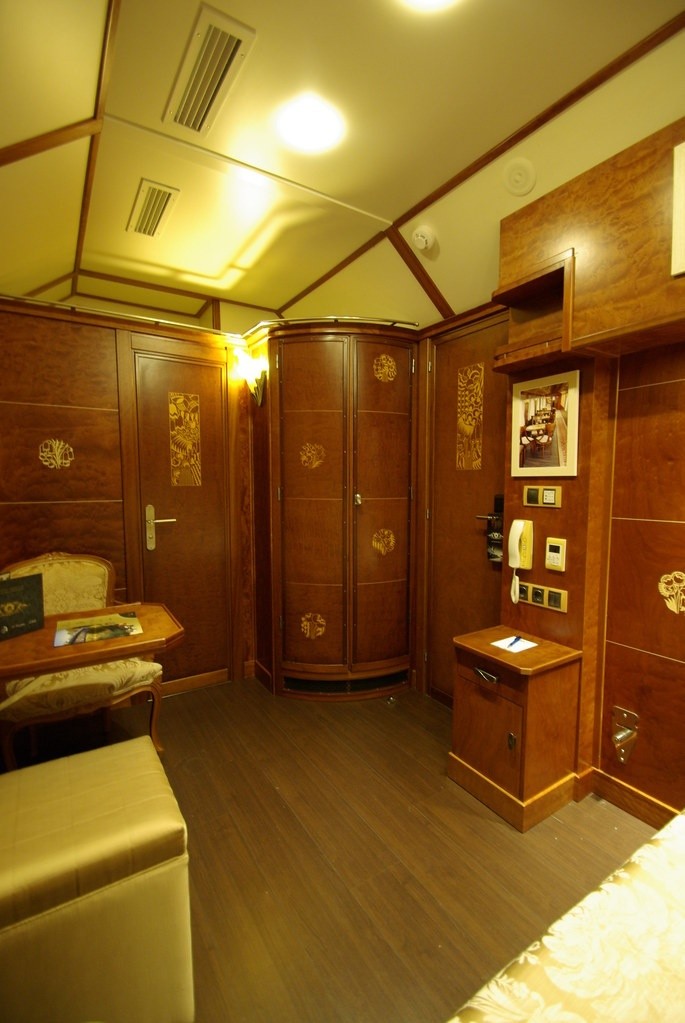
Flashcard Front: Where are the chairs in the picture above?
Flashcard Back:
[0,552,163,772]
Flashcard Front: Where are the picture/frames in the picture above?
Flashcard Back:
[511,370,580,477]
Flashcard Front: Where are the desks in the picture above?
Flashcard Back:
[0,603,186,683]
[0,740,194,1023]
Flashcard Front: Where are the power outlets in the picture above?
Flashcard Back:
[518,582,567,614]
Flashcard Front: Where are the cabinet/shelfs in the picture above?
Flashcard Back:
[448,626,581,834]
